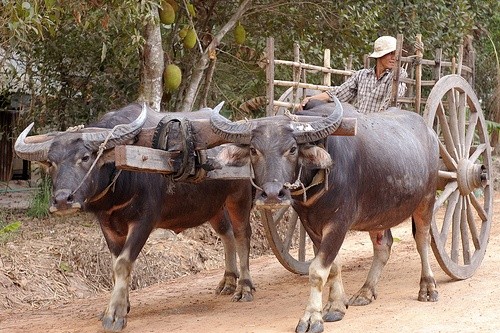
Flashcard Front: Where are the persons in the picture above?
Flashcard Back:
[300,35,407,113]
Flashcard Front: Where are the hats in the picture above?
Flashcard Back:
[368,35,396,57]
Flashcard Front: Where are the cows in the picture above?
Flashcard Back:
[13,98,260,331]
[208,91,440,333]
